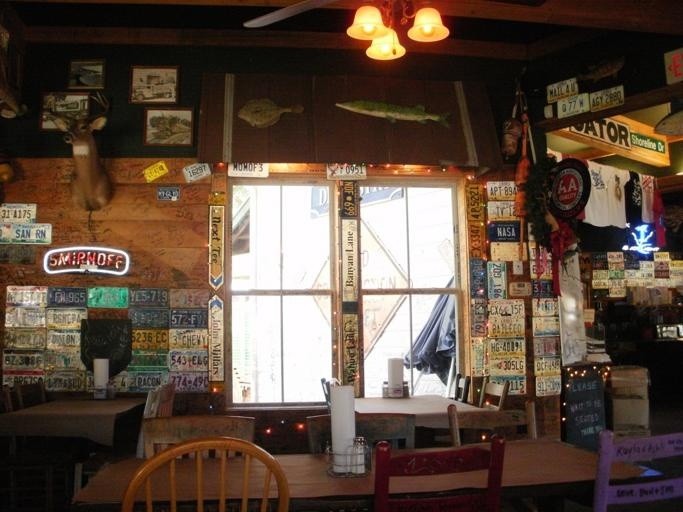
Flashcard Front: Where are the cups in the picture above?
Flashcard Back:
[345,435,371,478]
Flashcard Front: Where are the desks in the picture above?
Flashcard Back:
[71,441,662,510]
[1,396,147,448]
[355,395,478,428]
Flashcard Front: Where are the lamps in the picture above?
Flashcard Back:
[342,1,452,62]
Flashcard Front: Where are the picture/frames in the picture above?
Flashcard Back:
[37,57,196,150]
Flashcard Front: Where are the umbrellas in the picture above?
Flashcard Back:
[401,273,456,398]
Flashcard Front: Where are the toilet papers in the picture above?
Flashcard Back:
[388,357,404,396]
[93,358,108,399]
[331,384,355,475]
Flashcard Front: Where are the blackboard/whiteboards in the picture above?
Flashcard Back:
[80,318,132,379]
[562,362,606,452]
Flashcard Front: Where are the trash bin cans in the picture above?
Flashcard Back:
[610,366,652,437]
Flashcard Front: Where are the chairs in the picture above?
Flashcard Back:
[447,373,541,448]
[372,438,505,510]
[594,429,682,510]
[2,378,74,509]
[121,383,291,511]
[305,413,416,453]
